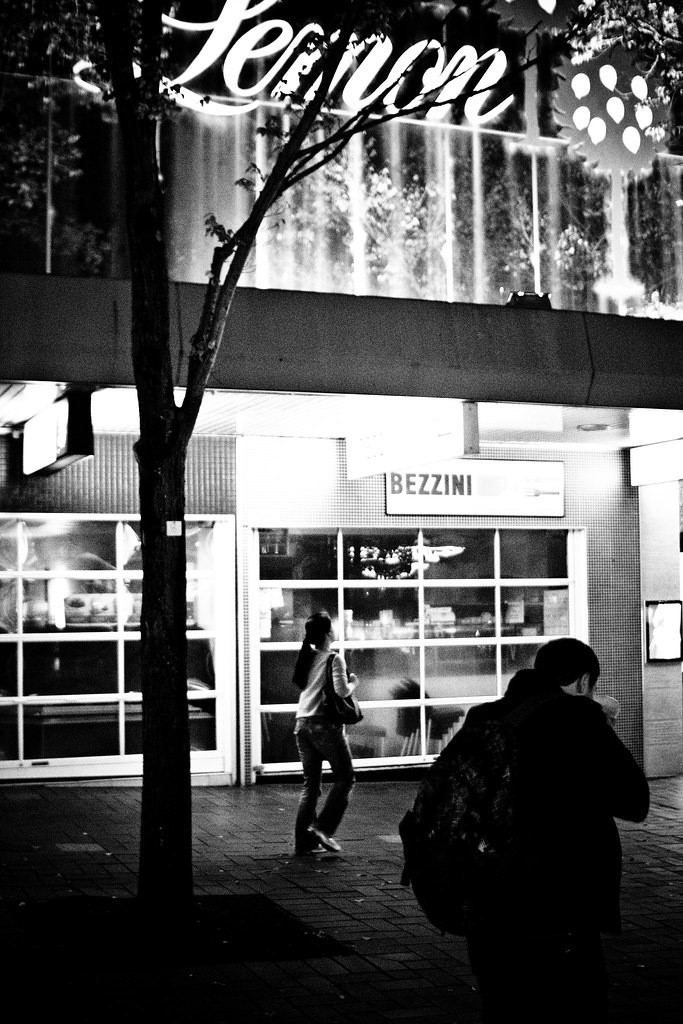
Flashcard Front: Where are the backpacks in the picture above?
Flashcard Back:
[398,809,483,939]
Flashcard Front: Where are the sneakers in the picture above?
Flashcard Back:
[307,825,342,852]
[295,843,328,854]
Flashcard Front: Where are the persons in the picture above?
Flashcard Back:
[465,638,652,1023]
[287,609,363,858]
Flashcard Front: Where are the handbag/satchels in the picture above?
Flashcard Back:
[322,653,363,724]
